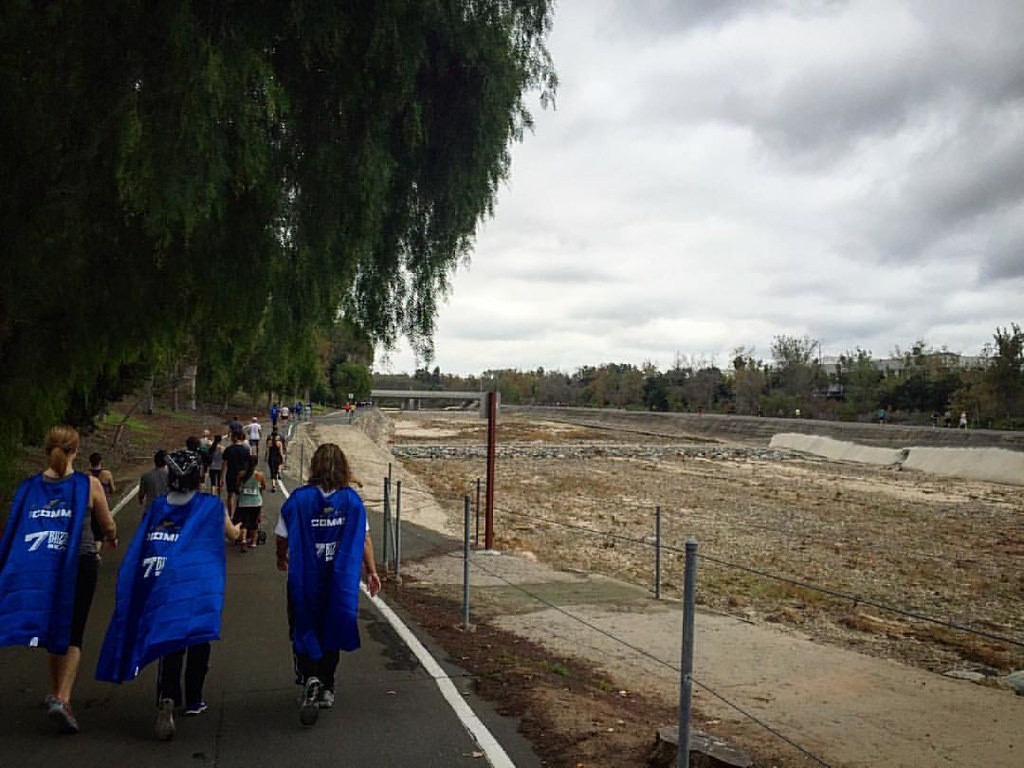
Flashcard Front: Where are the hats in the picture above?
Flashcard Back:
[252,417,257,421]
[203,429,210,435]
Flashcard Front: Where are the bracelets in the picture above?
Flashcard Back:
[105,536,118,542]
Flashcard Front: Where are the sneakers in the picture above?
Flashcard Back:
[185,701,207,714]
[319,689,335,708]
[299,676,322,725]
[44,694,79,735]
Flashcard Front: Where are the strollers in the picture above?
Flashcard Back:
[234,502,266,545]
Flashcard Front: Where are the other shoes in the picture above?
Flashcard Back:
[272,486,276,493]
[241,540,248,553]
[155,698,176,741]
[97,556,102,567]
[250,544,257,548]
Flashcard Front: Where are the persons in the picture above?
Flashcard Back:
[141,451,242,742]
[138,401,314,552]
[275,443,381,727]
[345,401,357,416]
[81,452,116,565]
[13,425,118,735]
[957,410,967,431]
[878,407,886,424]
[930,407,952,429]
